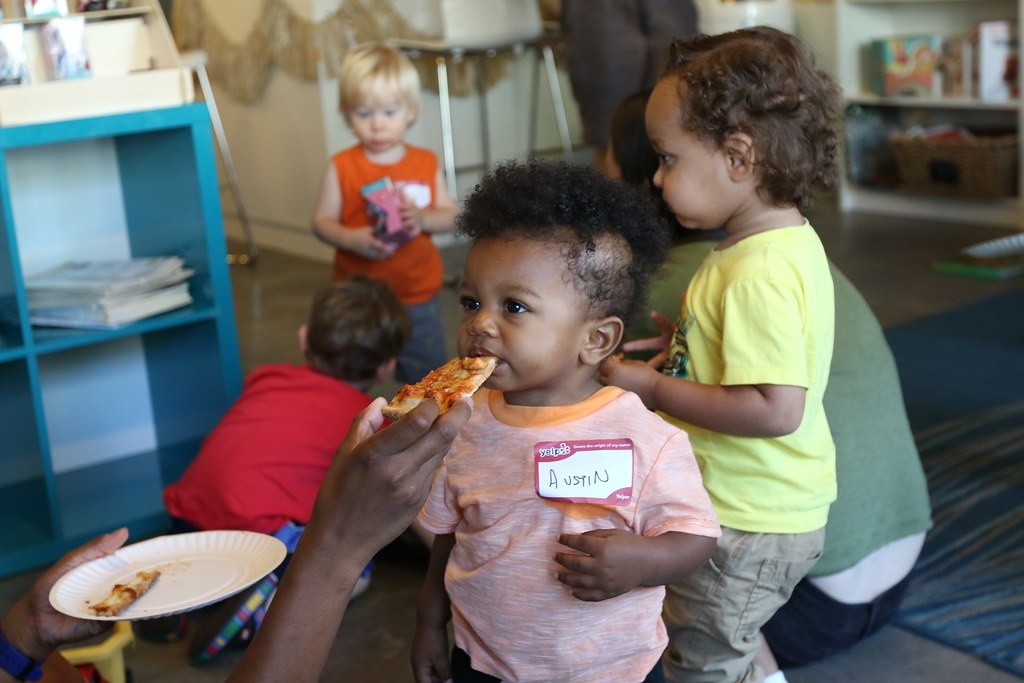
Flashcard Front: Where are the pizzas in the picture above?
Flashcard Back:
[380,354,497,420]
[87,569,159,617]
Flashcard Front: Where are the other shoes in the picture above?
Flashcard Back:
[187,571,278,665]
[132,610,190,643]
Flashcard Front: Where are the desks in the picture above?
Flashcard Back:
[387,22,576,236]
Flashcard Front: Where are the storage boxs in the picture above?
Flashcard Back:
[0,67,197,127]
[865,18,1017,100]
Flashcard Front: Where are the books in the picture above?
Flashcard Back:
[24,261,194,328]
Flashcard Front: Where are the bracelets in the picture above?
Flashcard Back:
[0,634,43,681]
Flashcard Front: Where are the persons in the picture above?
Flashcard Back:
[598,25,845,683]
[0,397,474,683]
[617,84,931,672]
[314,43,464,387]
[411,161,722,683]
[136,272,413,640]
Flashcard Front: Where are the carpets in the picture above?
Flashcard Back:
[879,281,1024,675]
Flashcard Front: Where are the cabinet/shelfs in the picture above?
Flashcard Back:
[790,0,1024,216]
[0,99,246,573]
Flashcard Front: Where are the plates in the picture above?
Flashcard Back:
[49,530,288,622]
